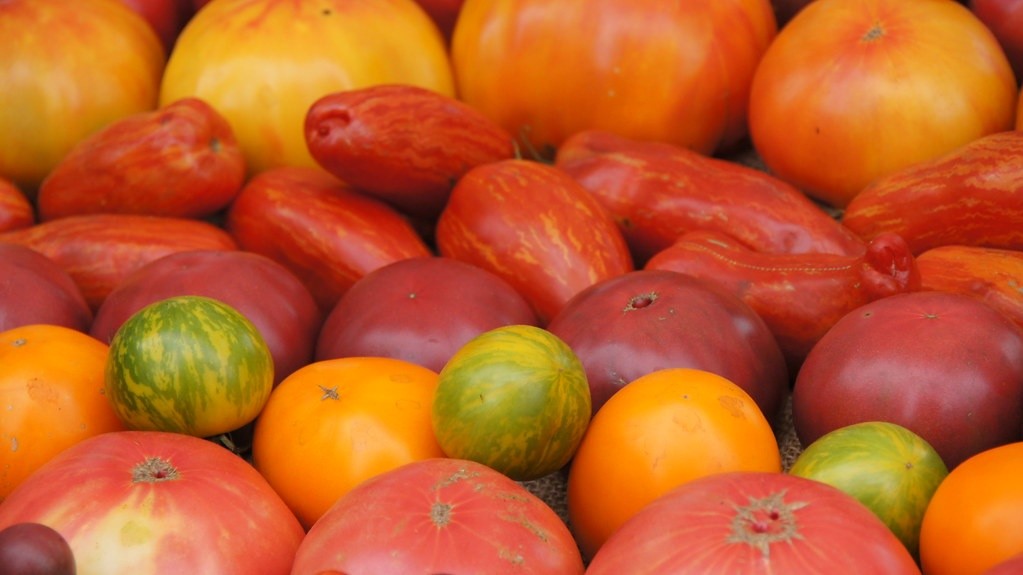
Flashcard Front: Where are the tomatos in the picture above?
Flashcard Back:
[0,0,1023,575]
